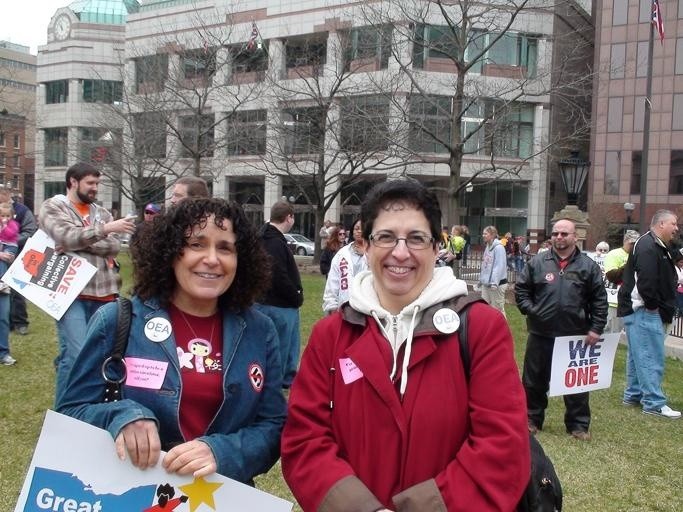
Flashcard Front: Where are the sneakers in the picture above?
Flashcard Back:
[527,422,539,435]
[0,354,17,366]
[623,397,640,407]
[19,326,28,335]
[641,403,682,421]
[565,427,593,442]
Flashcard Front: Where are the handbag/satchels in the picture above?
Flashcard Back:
[512,426,563,512]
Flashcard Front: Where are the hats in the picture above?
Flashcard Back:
[144,202,160,214]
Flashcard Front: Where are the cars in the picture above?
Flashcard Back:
[283,233,315,256]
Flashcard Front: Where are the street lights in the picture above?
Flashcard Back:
[624,202,635,232]
[461,179,474,269]
[549,147,594,252]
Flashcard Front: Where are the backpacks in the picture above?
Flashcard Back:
[499,238,509,246]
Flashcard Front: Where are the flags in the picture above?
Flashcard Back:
[651,0,666,47]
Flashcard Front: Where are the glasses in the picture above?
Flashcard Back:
[366,233,437,250]
[338,233,345,236]
[145,210,154,214]
[550,231,575,237]
